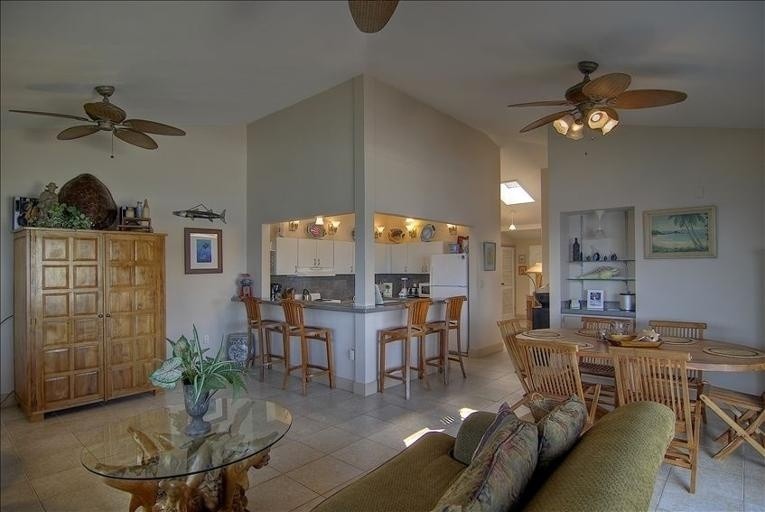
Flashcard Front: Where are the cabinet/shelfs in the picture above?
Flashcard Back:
[375,242,392,275]
[392,243,408,274]
[334,241,355,274]
[276,238,298,275]
[558,206,636,316]
[408,241,443,275]
[298,238,334,276]
[12,228,168,423]
[532,307,549,329]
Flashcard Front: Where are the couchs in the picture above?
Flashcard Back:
[311,400,677,512]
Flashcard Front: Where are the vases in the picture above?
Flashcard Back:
[226,332,255,371]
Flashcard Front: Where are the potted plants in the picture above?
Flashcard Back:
[147,321,249,435]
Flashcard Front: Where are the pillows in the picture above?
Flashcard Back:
[536,394,587,474]
[429,400,540,512]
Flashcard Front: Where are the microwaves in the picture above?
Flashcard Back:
[418,283,430,297]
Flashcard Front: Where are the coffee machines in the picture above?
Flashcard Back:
[270,283,281,300]
[398,277,410,296]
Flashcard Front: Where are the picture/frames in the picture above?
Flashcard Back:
[184,227,224,274]
[643,206,717,259]
[484,241,496,272]
[587,289,605,312]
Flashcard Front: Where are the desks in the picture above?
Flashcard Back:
[515,329,765,426]
[78,395,293,511]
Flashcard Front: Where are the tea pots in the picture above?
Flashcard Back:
[302,289,312,301]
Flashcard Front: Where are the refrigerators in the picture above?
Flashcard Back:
[429,253,470,356]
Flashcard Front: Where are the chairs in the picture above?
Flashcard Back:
[379,298,432,400]
[243,297,289,383]
[279,299,336,396]
[513,338,602,428]
[700,382,765,462]
[418,296,467,383]
[577,317,635,409]
[495,317,572,416]
[645,319,709,427]
[608,347,702,494]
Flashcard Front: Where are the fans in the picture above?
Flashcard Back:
[346,2,401,36]
[506,60,692,141]
[7,85,186,159]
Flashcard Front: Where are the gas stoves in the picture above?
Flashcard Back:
[314,298,342,303]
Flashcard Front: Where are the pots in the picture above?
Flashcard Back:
[618,293,638,311]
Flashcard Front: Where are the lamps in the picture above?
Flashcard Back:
[552,107,619,141]
[524,262,542,307]
[508,206,517,230]
[499,177,537,206]
[593,210,609,240]
[375,225,386,238]
[287,218,300,231]
[400,218,418,238]
[327,221,341,236]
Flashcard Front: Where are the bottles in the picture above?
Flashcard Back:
[136,200,143,218]
[572,237,580,261]
[126,207,134,218]
[143,199,150,218]
